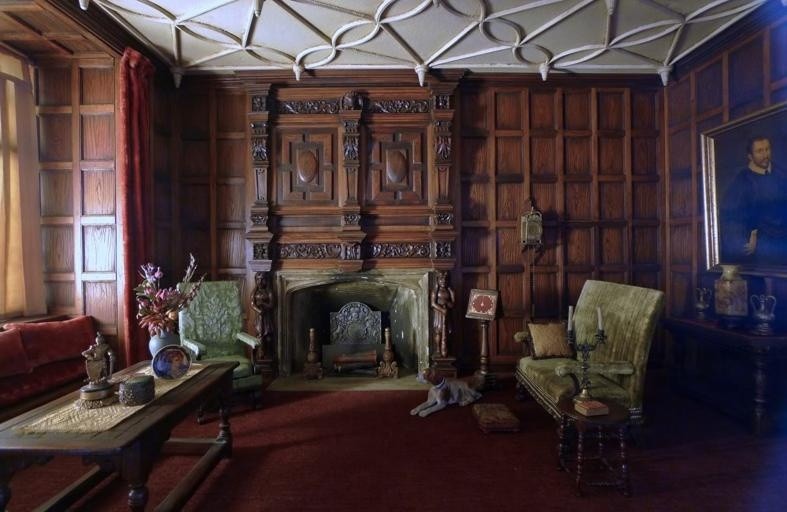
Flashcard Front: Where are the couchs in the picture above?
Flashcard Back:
[513,280,667,447]
[0,314,108,424]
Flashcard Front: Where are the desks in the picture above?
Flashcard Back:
[668,315,787,438]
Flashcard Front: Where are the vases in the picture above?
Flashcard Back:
[149,326,181,358]
[713,264,749,329]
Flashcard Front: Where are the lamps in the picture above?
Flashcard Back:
[519,205,542,252]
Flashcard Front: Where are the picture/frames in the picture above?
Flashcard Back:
[699,103,787,280]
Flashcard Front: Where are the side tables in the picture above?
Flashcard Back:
[558,398,631,497]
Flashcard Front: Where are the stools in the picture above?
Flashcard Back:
[472,403,520,435]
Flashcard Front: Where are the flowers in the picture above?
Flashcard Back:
[132,252,208,338]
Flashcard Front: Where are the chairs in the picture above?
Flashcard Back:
[177,281,273,425]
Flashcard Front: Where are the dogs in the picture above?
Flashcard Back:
[407,361,496,418]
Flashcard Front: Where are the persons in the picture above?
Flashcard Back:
[717,135,786,268]
[428,268,454,331]
[247,272,276,337]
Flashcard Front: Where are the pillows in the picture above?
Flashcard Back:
[0,327,33,377]
[524,318,573,361]
[0,316,97,368]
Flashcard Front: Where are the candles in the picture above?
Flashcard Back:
[567,305,574,332]
[596,306,603,331]
